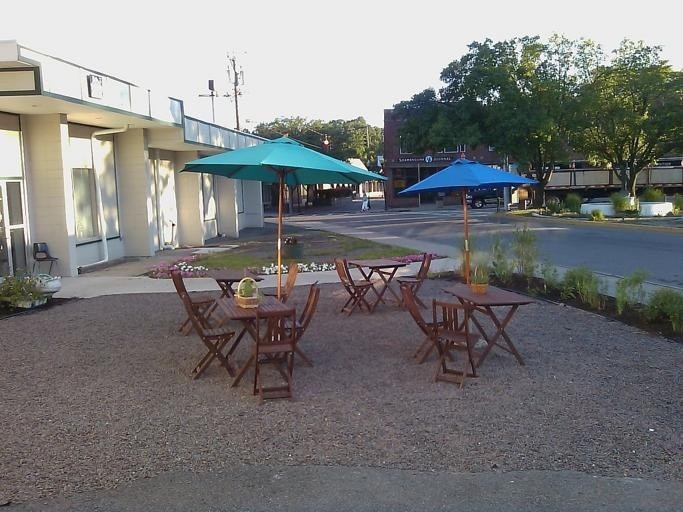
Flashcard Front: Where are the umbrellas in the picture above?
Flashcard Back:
[397,154,539,285]
[177,131,387,300]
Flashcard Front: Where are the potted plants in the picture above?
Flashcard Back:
[469,264,491,296]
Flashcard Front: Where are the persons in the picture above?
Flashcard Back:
[359,190,369,213]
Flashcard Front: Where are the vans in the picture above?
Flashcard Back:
[313,188,353,207]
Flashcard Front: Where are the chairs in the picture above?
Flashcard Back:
[171,261,320,406]
[335,243,480,390]
[33,243,59,275]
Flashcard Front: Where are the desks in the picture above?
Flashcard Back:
[442,284,537,368]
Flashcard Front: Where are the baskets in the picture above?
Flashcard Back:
[234,276,261,309]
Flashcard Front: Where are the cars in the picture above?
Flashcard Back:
[465,186,532,209]
